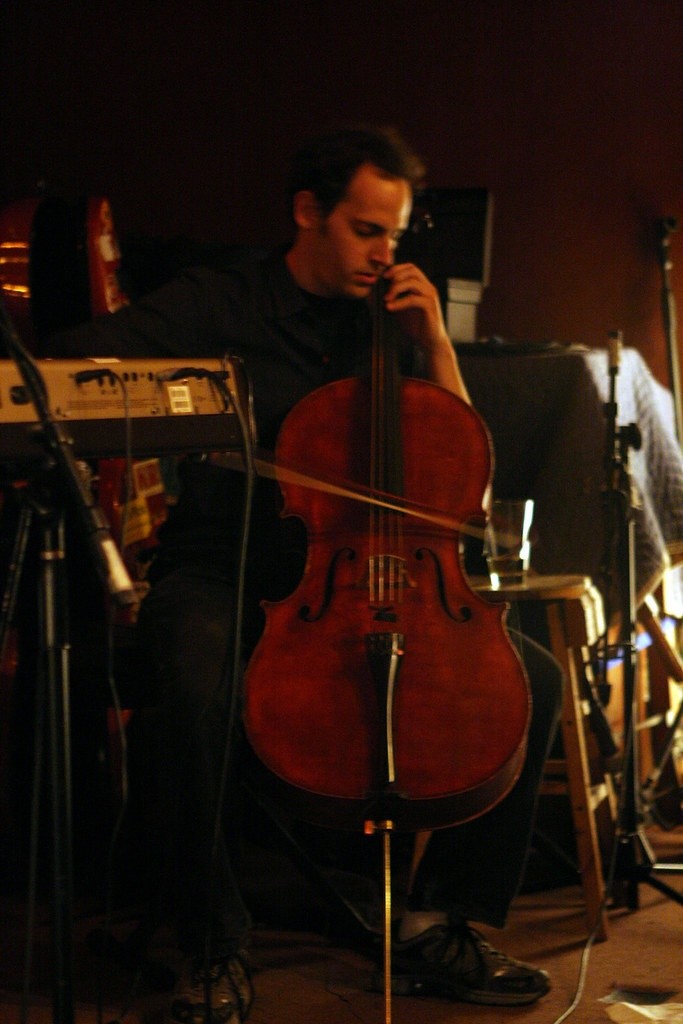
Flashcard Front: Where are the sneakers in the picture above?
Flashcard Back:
[372,918,552,1005]
[172,942,253,1013]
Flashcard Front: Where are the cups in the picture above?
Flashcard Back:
[485,499,534,585]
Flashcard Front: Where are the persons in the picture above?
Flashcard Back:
[49,122,555,1024]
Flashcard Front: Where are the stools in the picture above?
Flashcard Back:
[470,570,622,937]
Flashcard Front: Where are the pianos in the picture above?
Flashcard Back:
[0,351,258,1014]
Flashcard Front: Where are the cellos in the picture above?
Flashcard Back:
[237,275,534,1024]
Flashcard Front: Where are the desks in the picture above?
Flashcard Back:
[457,345,683,622]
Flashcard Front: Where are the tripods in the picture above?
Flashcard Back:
[573,421,683,913]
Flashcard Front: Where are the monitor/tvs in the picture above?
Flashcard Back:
[395,183,493,286]
